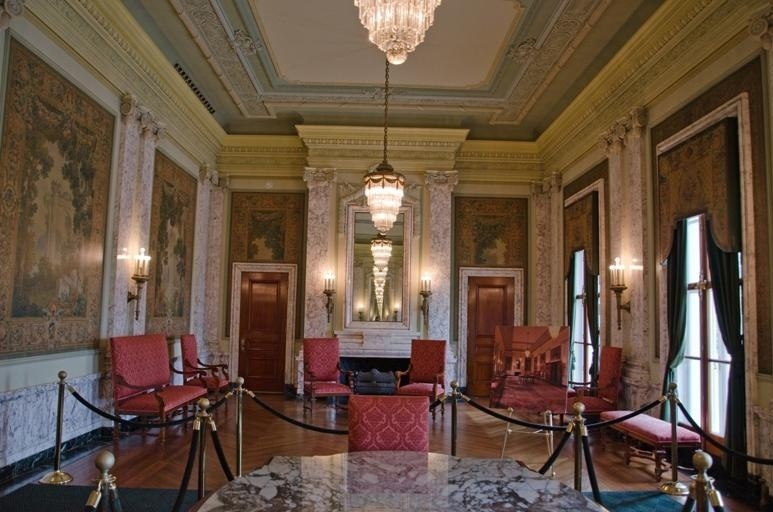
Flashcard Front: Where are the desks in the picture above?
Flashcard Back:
[196,451,609,512]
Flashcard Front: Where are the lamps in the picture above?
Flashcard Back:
[363,59,406,235]
[323,279,336,323]
[609,258,630,329]
[371,232,392,320]
[420,280,433,324]
[127,248,152,320]
[354,0,442,65]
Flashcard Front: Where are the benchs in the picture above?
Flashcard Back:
[110,334,209,445]
[600,411,701,482]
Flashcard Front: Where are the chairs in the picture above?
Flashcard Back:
[181,334,229,412]
[303,338,358,416]
[560,346,623,426]
[348,395,430,451]
[394,339,447,420]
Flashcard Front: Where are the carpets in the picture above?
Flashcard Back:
[0,483,206,512]
[582,491,695,512]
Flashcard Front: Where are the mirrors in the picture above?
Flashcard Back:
[345,205,414,329]
